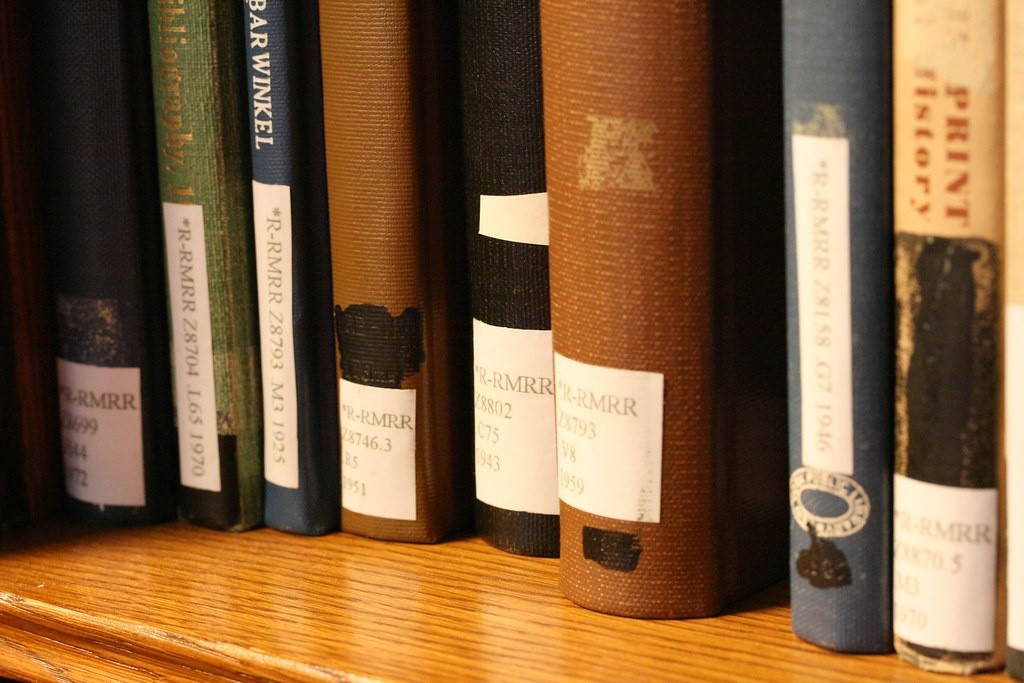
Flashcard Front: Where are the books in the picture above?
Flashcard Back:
[1,1,1023,683]
